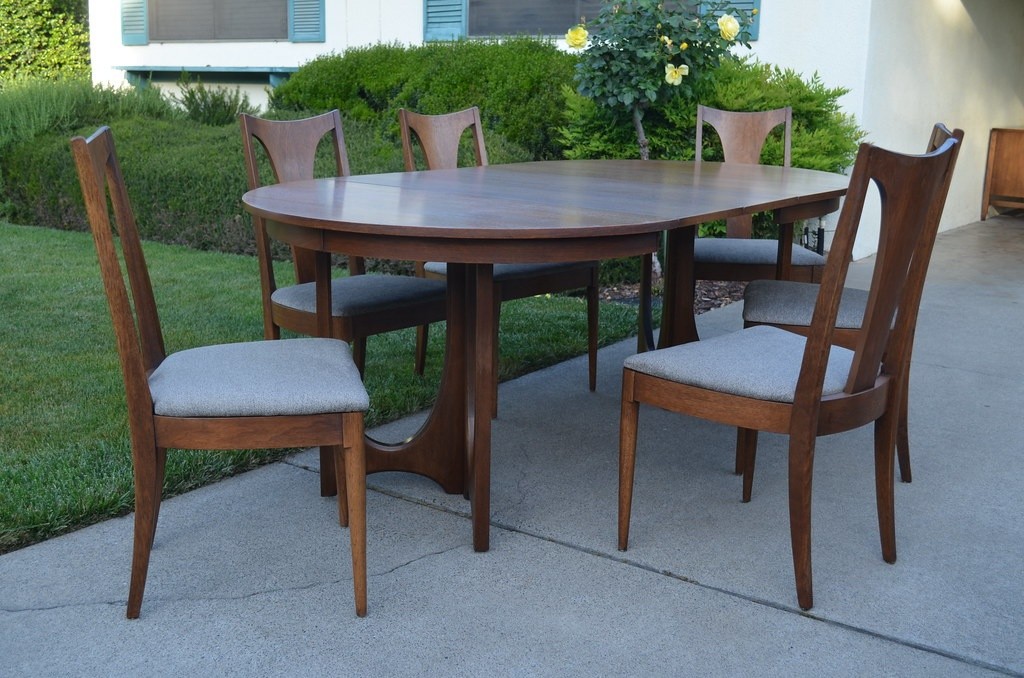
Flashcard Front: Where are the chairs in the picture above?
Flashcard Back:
[617,138,962,611]
[396,105,601,420]
[692,104,827,300]
[70,124,372,621]
[237,107,449,383]
[736,121,964,504]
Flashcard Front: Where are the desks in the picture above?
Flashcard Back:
[243,158,851,552]
[980,128,1024,222]
